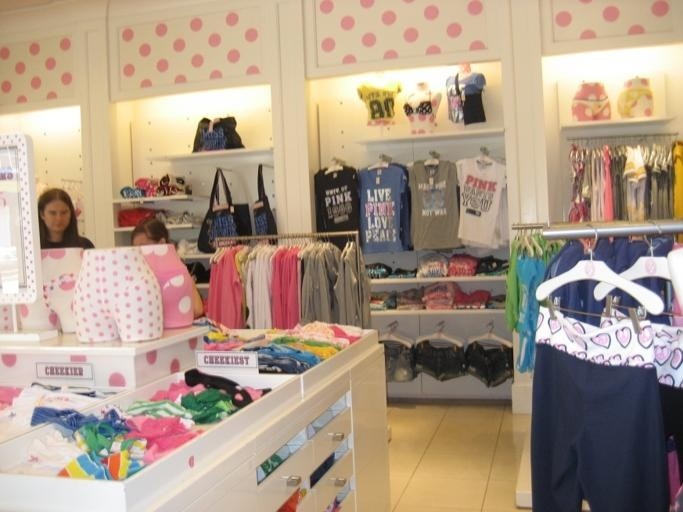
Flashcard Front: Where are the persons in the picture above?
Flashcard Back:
[36,189,95,249]
[130,218,202,318]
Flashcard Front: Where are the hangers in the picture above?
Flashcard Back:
[209,232,358,265]
[568,133,678,152]
[377,319,511,351]
[512,227,682,318]
[323,145,492,174]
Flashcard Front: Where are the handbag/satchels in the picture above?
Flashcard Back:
[253,164,276,245]
[192,117,245,152]
[197,168,252,254]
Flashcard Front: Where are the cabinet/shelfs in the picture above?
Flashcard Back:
[519,35,682,226]
[283,49,519,398]
[94,70,283,292]
